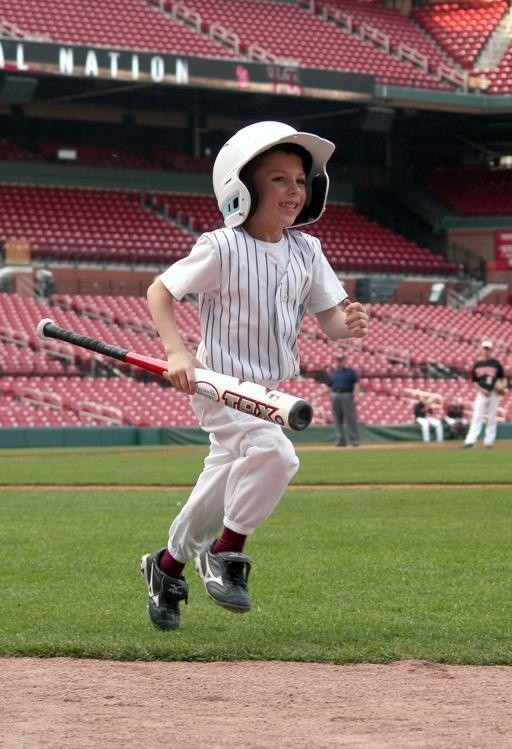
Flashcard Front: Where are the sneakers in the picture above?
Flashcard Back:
[140,547,189,631]
[193,539,252,613]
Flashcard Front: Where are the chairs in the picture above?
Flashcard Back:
[0,186,512,279]
[2,293,511,432]
[2,1,512,96]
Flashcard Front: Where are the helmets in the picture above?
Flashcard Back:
[212,120,337,230]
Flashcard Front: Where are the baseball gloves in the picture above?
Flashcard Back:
[495,376,508,393]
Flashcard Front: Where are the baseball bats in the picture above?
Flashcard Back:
[37,318,313,432]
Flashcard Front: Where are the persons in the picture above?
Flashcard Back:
[462,341,507,451]
[411,395,445,444]
[444,397,470,440]
[135,119,370,630]
[326,351,364,449]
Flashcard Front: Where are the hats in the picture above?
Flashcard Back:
[482,341,493,349]
